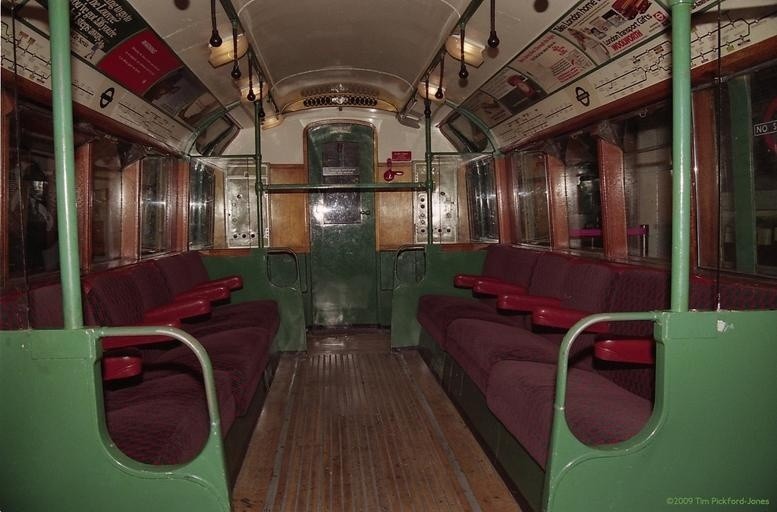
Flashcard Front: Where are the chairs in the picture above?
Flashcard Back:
[10,250,280,468]
[415,243,777,471]
